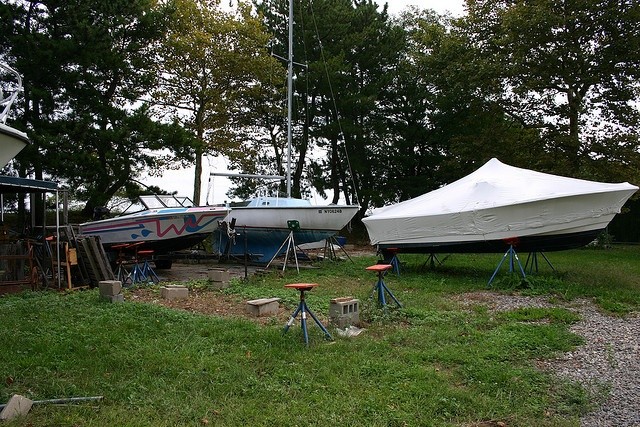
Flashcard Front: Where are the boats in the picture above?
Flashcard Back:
[361,157,639,253]
[79,196,232,253]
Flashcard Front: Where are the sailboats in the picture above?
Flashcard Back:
[210,0,359,246]
